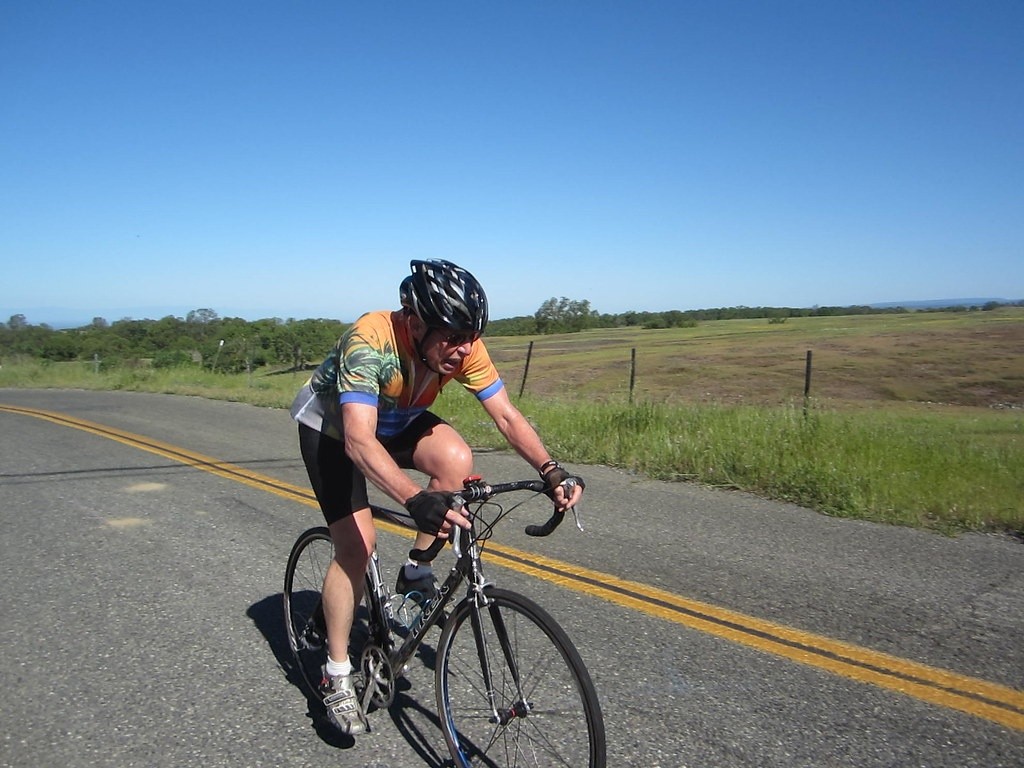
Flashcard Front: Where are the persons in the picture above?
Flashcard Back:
[290,258,585,739]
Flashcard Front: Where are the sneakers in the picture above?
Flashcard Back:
[395,565,456,630]
[318,663,367,736]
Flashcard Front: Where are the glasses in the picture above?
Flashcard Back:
[431,325,484,347]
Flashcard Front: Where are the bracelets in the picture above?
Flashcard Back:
[539,461,560,478]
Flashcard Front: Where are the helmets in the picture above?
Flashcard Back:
[399,257,489,333]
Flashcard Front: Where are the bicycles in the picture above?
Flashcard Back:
[283,474,607,768]
